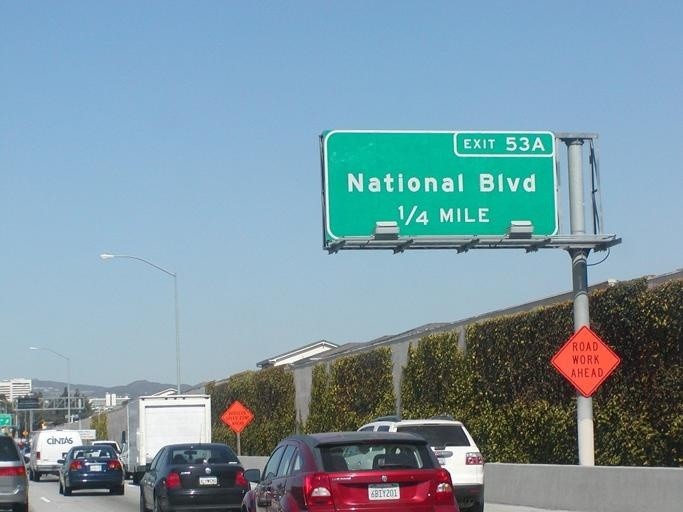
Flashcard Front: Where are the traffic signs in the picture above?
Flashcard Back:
[321,128,558,244]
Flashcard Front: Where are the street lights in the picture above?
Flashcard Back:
[97,254,183,397]
[28,345,72,430]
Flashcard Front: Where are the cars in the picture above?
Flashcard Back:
[0,436,29,512]
[137,441,248,510]
[92,440,124,457]
[242,434,457,512]
[61,445,124,493]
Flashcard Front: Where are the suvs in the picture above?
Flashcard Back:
[359,412,483,512]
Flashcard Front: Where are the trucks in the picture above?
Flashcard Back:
[32,430,82,480]
